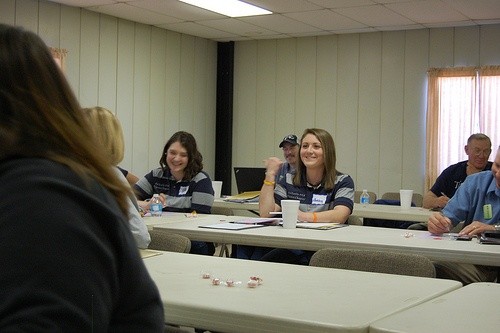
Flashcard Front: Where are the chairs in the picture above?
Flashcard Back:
[148,229,192,254]
[309,191,436,278]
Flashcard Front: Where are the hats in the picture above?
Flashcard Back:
[278,134,301,148]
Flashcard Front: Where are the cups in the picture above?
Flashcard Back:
[400,189,413,208]
[212,181,222,201]
[281,199,301,229]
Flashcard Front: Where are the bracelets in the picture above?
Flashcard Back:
[263,180,275,185]
[314,212,316,223]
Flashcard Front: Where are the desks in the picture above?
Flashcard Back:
[348,203,439,222]
[141,248,463,333]
[369,283,500,332]
[211,195,260,215]
[154,216,500,283]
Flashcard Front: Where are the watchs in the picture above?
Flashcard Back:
[494,225,500,230]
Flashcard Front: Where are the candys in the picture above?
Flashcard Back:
[202,273,262,288]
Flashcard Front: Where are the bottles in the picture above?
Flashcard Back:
[360,189,370,209]
[151,194,163,217]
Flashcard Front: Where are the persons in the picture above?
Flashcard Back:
[131,130,216,256]
[236,128,355,266]
[428,145,500,286]
[0,23,164,333]
[407,133,495,236]
[275,134,300,181]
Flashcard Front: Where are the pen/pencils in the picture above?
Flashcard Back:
[229,222,255,225]
[441,192,450,200]
[437,206,452,229]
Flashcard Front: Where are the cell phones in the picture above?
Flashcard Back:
[457,236,471,240]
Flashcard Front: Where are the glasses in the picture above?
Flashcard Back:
[164,150,189,158]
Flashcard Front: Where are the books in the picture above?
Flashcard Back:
[480,233,500,245]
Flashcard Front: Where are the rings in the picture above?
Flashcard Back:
[474,226,476,228]
